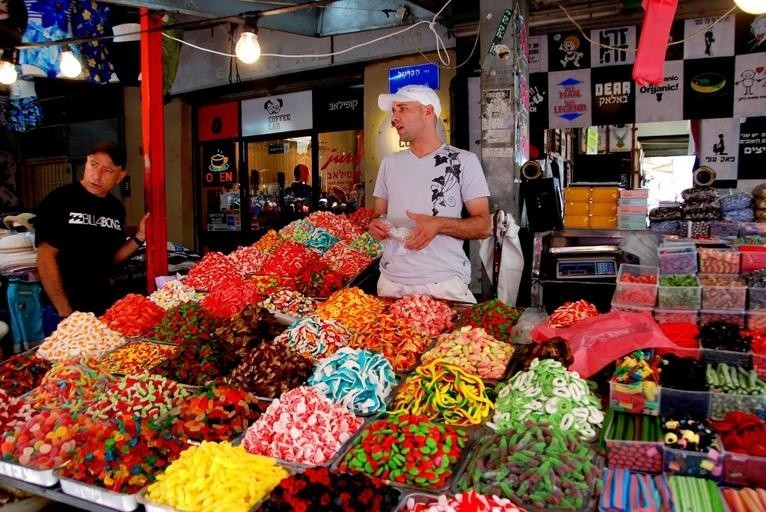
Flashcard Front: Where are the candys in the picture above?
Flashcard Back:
[0,180,766,512]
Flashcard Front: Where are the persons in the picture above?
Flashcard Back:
[367,83,493,304]
[33,136,150,338]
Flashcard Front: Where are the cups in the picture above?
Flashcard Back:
[295,202,303,213]
[210,154,228,168]
[283,198,290,206]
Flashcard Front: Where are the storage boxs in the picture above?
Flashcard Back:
[601,241,766,484]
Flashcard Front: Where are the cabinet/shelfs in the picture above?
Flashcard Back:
[563,181,622,230]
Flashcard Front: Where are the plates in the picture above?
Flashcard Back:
[208,162,229,172]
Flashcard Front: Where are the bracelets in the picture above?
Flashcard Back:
[132,235,144,246]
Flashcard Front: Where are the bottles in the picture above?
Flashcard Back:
[222,208,226,223]
[261,201,281,211]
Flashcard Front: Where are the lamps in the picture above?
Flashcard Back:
[58,39,82,79]
[0,46,18,85]
[234,10,264,65]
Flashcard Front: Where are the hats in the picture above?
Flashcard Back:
[0,213,38,276]
[378,84,441,120]
[86,140,126,172]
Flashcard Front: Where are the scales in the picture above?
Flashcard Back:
[548,245,618,280]
